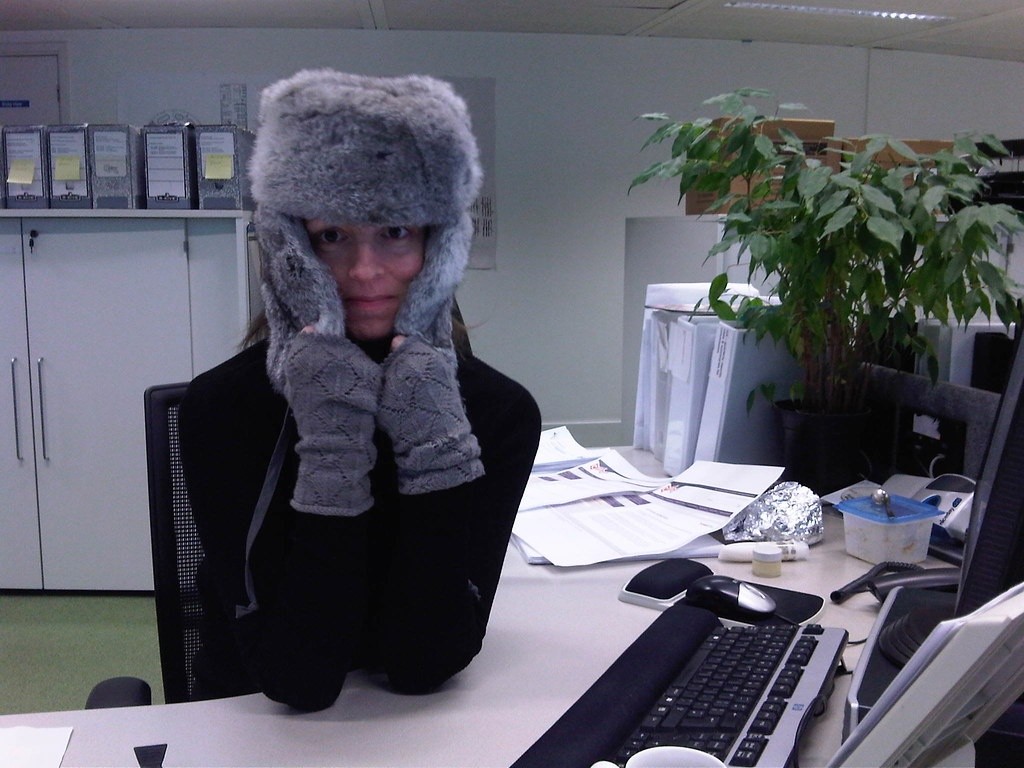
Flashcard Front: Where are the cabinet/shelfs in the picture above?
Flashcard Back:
[0,209,235,592]
[625,214,1024,446]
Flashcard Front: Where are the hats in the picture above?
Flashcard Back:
[247,69,484,387]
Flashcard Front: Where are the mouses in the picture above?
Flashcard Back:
[687,576,777,621]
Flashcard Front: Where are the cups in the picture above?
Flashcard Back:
[591,746,727,767]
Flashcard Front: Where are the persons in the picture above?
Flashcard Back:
[178,70,542,712]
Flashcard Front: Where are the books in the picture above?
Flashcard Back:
[510,532,726,564]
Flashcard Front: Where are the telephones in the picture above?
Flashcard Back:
[868,559,961,604]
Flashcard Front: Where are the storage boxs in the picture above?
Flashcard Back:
[0,122,256,210]
[685,117,956,214]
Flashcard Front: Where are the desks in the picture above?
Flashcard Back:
[0,442,1024,768]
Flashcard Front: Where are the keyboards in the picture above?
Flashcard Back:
[605,622,849,768]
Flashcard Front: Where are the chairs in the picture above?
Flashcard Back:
[83,382,209,710]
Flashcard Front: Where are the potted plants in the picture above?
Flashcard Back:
[626,80,1024,506]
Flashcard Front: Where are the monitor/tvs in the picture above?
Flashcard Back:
[876,332,1024,672]
